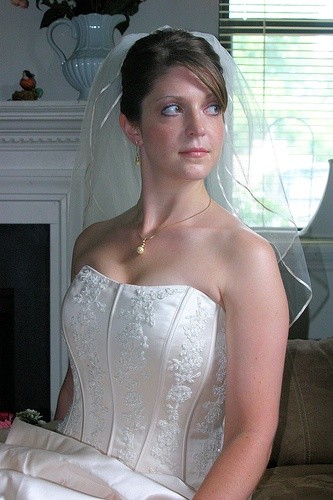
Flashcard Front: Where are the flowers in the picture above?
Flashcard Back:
[0,409,47,429]
[10,0,147,35]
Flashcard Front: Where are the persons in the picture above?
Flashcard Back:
[0,24,313,500]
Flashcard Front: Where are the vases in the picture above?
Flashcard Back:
[46,13,126,103]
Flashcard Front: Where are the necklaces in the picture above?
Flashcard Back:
[135,196,211,255]
